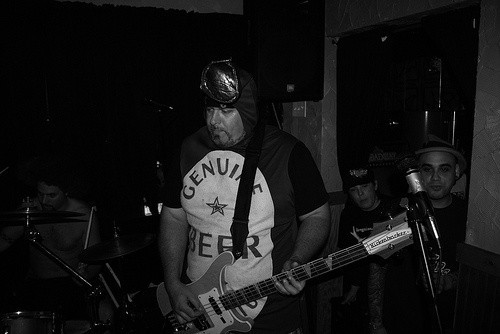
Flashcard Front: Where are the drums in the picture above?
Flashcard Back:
[0,309,61,334]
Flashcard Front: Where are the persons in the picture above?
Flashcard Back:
[336,165,409,334]
[0,172,116,334]
[158,58,331,334]
[365,151,469,334]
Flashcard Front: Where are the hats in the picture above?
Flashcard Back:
[413,134,468,174]
[343,163,373,190]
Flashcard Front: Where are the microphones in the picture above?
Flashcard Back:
[396,147,441,239]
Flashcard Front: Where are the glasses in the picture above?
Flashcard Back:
[422,166,453,174]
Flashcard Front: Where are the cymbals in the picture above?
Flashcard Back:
[0,210,86,217]
[81,230,155,262]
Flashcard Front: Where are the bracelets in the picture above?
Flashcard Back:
[368,322,384,331]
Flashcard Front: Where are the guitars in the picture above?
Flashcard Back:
[155,199,442,334]
